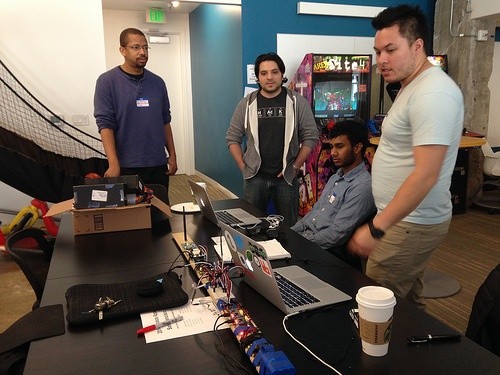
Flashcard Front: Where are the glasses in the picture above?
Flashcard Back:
[127,45,151,49]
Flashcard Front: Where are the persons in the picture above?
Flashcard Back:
[290,120,377,251]
[94,27,178,192]
[348,5,465,310]
[225,53,320,228]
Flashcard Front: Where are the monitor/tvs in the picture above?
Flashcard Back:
[427,55,448,72]
[311,73,361,118]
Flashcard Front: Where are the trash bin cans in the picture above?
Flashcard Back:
[449,166,467,215]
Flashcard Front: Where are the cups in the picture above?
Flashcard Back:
[355,286,397,357]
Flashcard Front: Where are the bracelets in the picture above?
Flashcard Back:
[293,165,297,169]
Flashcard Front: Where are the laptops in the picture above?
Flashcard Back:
[187,177,262,227]
[218,220,352,314]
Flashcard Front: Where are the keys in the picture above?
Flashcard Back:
[94,302,107,320]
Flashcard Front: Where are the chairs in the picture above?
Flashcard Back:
[5,228,53,311]
[31,199,59,236]
[472,137,500,214]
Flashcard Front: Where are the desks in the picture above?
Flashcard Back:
[369,135,487,216]
[23,199,500,375]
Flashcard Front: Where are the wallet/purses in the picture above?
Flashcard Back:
[64,271,189,327]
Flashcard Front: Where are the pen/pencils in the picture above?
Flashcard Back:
[137,316,183,335]
[406,333,461,343]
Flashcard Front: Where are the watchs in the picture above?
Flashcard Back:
[368,222,384,238]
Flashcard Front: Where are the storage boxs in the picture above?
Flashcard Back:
[42,195,171,235]
